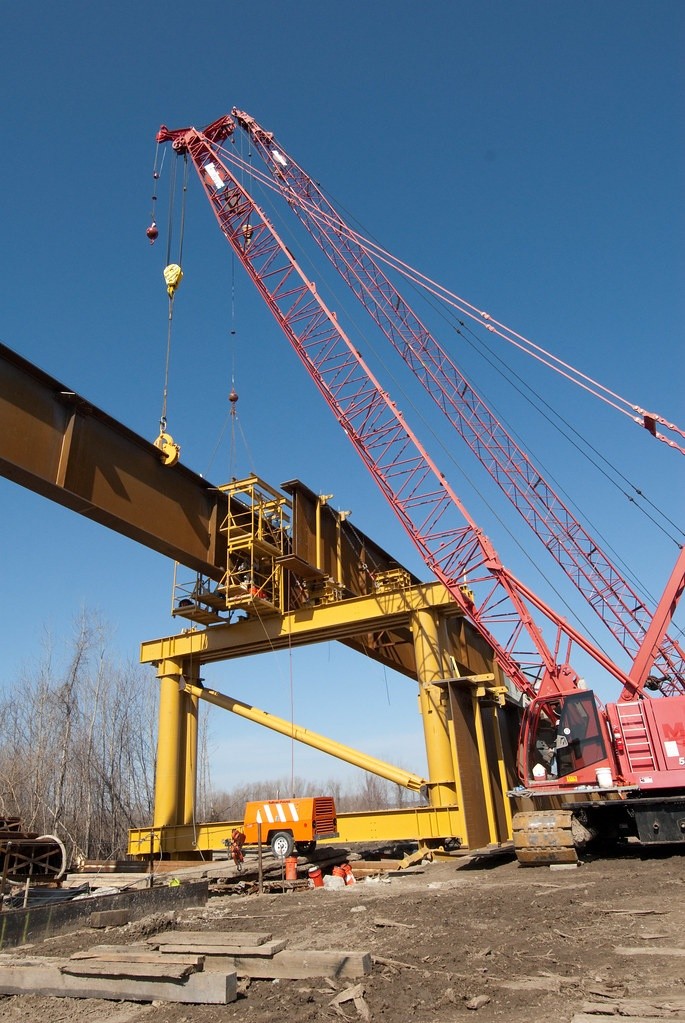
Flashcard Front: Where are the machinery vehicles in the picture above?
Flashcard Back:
[144,107,685,865]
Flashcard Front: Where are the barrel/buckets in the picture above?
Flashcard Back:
[595,769,613,788]
